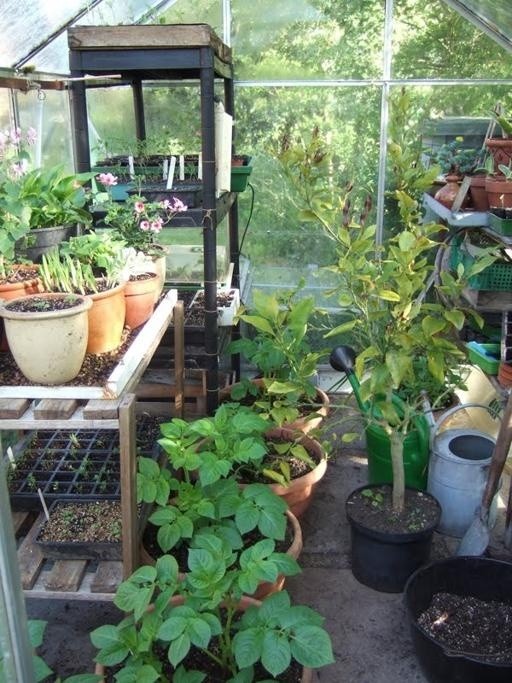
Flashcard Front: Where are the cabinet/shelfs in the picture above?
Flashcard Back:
[0,286,189,620]
[65,47,241,416]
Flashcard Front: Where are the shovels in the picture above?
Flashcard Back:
[456,389,512,558]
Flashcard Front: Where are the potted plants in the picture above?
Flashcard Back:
[6,171,84,258]
[49,250,131,359]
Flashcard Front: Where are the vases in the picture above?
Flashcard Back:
[1,256,43,304]
[0,293,92,385]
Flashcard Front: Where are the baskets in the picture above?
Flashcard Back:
[448,234,512,292]
